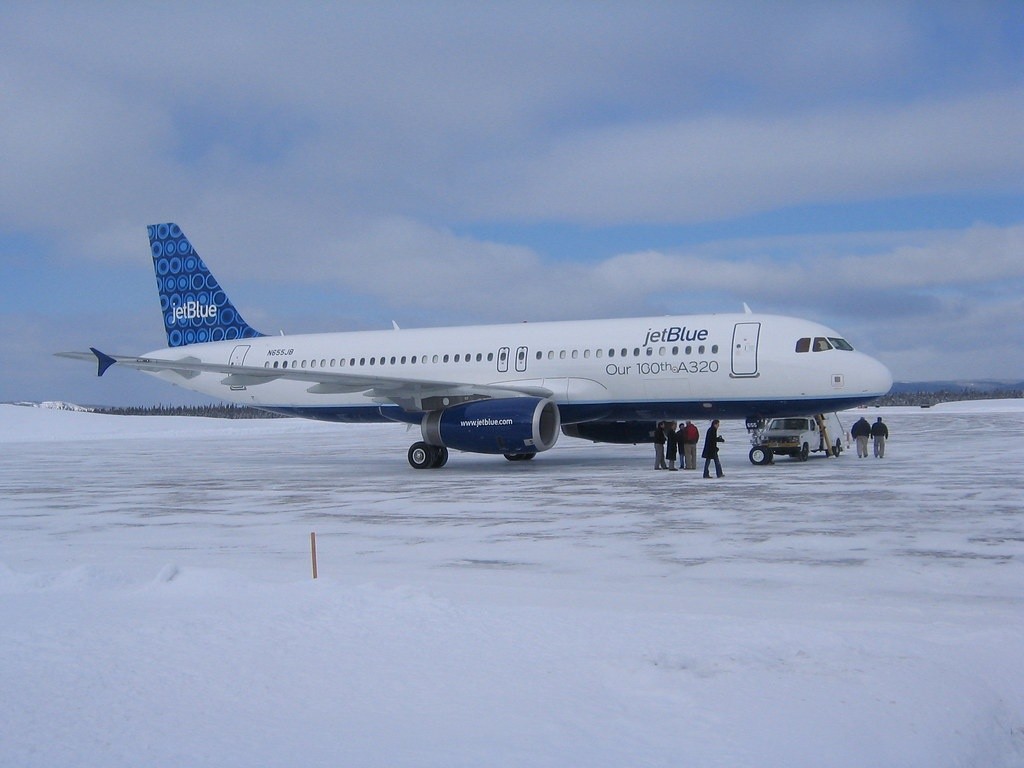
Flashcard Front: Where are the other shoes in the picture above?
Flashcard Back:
[875,455,884,458]
[864,454,868,457]
[716,475,726,478]
[684,468,696,470]
[662,467,671,469]
[654,468,662,470]
[703,475,713,479]
[669,468,678,471]
[859,454,863,458]
[678,466,684,469]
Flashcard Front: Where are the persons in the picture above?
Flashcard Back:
[701,419,725,478]
[653,421,669,470]
[871,416,889,459]
[851,416,872,458]
[683,420,700,469]
[676,422,686,468]
[665,421,678,471]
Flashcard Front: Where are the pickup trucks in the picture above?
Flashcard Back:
[759,417,850,459]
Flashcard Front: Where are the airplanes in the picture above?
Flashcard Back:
[49,220,896,471]
